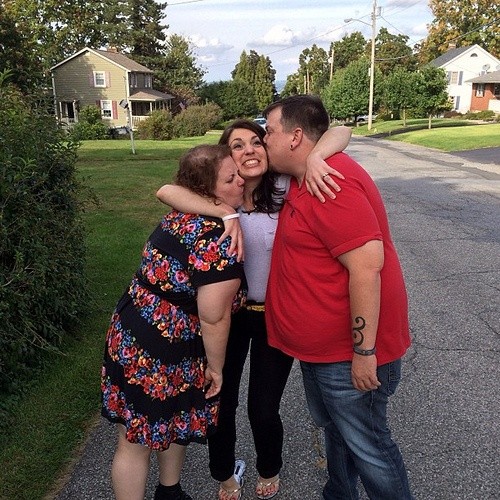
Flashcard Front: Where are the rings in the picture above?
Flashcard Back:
[322,173,328,178]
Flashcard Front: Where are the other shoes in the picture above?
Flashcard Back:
[153,487,194,500]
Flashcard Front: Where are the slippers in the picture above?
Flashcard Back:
[216,460,246,500]
[255,474,280,500]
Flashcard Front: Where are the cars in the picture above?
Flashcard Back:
[253,116,267,132]
[356,112,380,122]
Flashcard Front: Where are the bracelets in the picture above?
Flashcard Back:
[353,346,376,356]
[222,213,240,221]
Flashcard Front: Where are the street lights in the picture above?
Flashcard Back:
[344,17,378,130]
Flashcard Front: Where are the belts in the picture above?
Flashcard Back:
[241,303,265,312]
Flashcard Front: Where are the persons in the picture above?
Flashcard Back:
[155,120,352,500]
[262,95,414,500]
[100,143,245,500]
